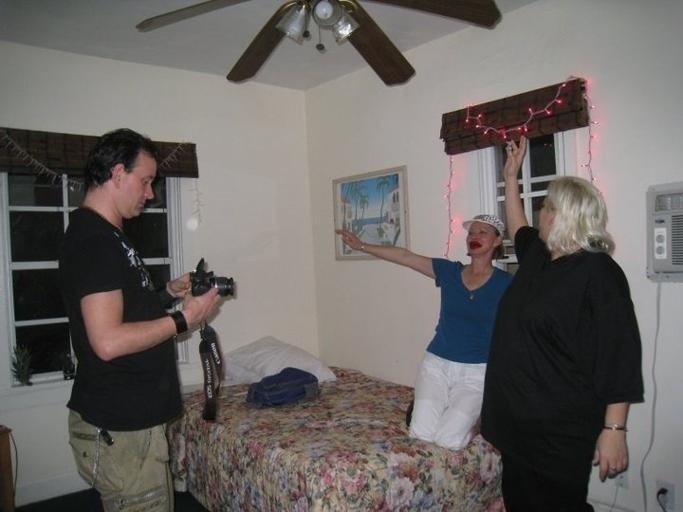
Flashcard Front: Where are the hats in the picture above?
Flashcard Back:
[462,214,505,240]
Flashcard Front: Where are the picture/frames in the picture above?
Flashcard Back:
[332,165,409,260]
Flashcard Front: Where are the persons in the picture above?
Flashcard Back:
[331,213,515,451]
[478,133,644,511]
[55,127,222,511]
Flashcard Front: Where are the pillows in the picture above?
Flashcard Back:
[222,336,337,388]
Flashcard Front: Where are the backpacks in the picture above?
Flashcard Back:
[246,366,320,409]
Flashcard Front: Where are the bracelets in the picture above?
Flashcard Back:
[358,241,366,250]
[602,424,628,430]
[167,310,188,336]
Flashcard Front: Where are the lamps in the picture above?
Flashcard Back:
[280,0,356,52]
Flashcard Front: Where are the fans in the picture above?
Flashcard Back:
[134,0,503,86]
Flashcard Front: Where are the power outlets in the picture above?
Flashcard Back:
[654,478,675,511]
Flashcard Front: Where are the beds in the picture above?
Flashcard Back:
[166,366,502,512]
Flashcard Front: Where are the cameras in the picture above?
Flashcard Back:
[189,258,234,298]
